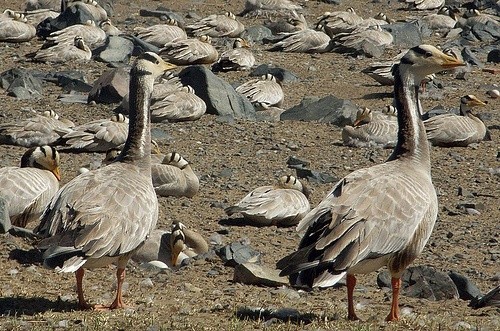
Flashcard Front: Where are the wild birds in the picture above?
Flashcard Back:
[342,108,398,148]
[424,96,489,146]
[62,114,131,152]
[221,174,311,227]
[275,41,471,323]
[0,145,61,234]
[33,51,181,312]
[132,219,208,271]
[150,153,201,197]
[0,109,74,146]
[2,0,499,121]
